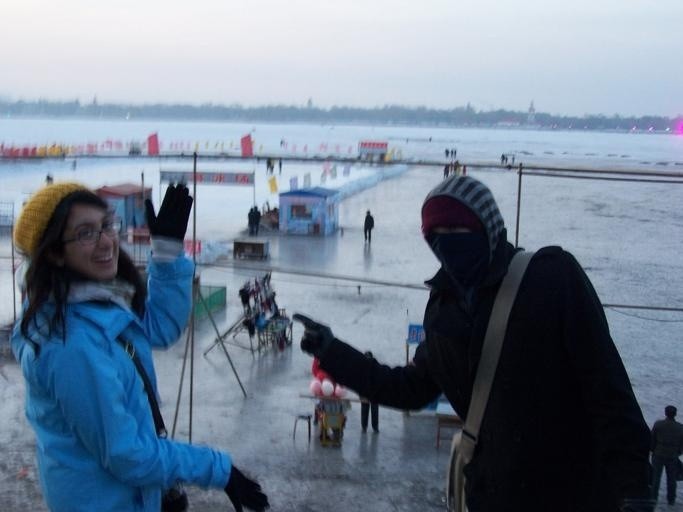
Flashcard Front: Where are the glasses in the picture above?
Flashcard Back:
[64,218,123,247]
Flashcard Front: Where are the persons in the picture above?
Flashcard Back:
[358,350,379,433]
[266,157,282,175]
[8,184,271,511]
[253,206,260,235]
[443,148,467,177]
[363,209,374,241]
[248,208,255,235]
[648,405,682,504]
[47,173,53,186]
[293,175,657,511]
[499,151,504,165]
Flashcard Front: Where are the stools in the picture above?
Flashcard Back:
[292,414,312,440]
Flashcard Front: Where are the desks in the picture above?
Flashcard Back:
[233,239,268,260]
[300,382,368,425]
[434,402,463,448]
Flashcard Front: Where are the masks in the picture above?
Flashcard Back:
[424,233,488,279]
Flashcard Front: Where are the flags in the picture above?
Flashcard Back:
[147,133,159,156]
[280,138,351,155]
[169,141,233,150]
[269,162,351,195]
[240,133,252,158]
[0,137,146,157]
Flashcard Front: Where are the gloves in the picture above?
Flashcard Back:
[293,313,333,360]
[226,465,270,512]
[144,183,193,241]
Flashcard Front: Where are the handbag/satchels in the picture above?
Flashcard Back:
[677,458,683,481]
[445,429,477,512]
[155,426,190,512]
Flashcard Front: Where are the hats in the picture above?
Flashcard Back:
[12,182,85,257]
[422,196,474,231]
